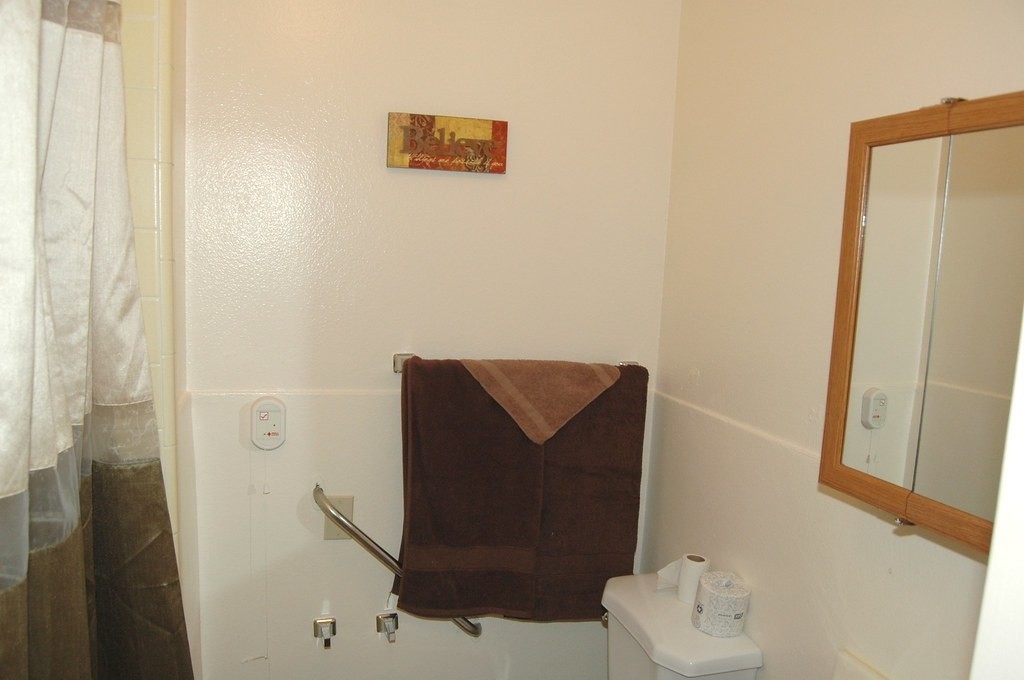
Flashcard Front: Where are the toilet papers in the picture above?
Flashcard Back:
[655,552,751,638]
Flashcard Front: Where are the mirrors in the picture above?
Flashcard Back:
[818,89,1024,553]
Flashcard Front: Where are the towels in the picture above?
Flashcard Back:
[389,355,650,625]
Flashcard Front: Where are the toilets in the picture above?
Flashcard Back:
[600,572,763,680]
